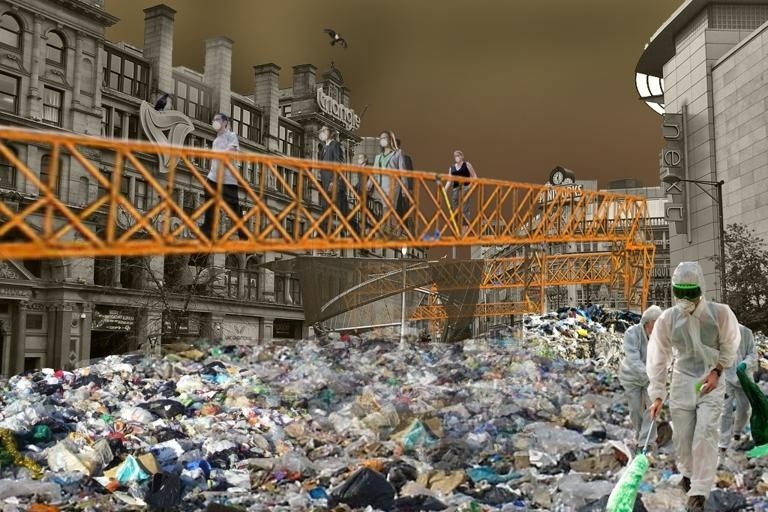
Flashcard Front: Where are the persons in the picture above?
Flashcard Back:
[441,149,479,236]
[393,137,417,236]
[317,124,370,258]
[356,151,371,197]
[616,302,665,443]
[643,260,742,511]
[719,320,760,468]
[364,129,410,257]
[194,112,251,243]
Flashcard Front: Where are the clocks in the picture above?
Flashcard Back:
[552,171,564,184]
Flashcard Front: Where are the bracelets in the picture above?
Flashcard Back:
[711,367,722,377]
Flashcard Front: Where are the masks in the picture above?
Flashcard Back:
[358,160,364,164]
[212,121,222,130]
[319,133,328,141]
[455,157,460,163]
[379,139,388,146]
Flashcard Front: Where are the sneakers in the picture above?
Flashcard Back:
[679,477,704,512]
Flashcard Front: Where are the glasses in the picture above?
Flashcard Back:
[672,284,701,299]
